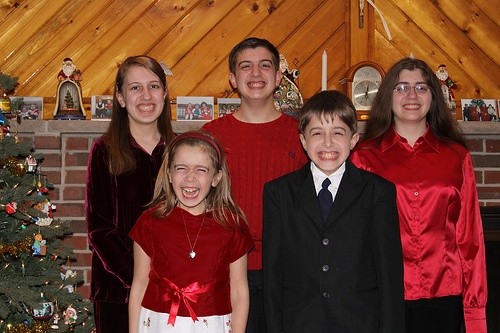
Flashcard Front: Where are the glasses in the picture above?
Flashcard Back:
[394,85,431,94]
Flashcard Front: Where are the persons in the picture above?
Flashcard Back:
[57,58,83,86]
[197,37,309,333]
[19,104,39,120]
[463,104,496,122]
[273,54,304,113]
[96,98,113,119]
[219,104,240,117]
[185,102,213,120]
[262,89,405,333]
[127,130,257,333]
[436,64,459,110]
[84,54,176,333]
[351,57,488,333]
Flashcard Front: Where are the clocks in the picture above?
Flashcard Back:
[338,60,386,121]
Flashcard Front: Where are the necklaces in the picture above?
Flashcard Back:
[178,202,206,259]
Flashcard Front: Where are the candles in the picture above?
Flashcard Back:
[322,48,327,91]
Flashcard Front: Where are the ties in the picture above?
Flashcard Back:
[318,178,333,224]
